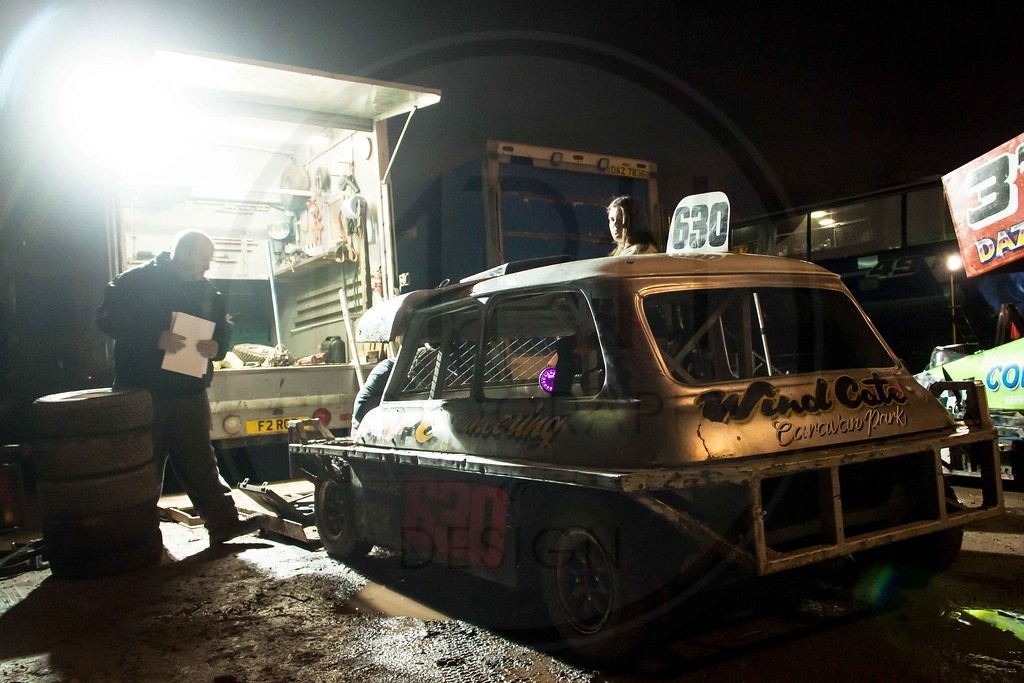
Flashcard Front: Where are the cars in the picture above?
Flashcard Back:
[289,249,1005,656]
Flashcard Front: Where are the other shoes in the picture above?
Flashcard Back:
[209,513,270,544]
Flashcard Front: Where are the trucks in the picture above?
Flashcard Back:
[92,48,441,485]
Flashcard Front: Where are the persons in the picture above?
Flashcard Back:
[979,306,998,344]
[604,195,660,258]
[351,348,438,437]
[94,231,266,544]
